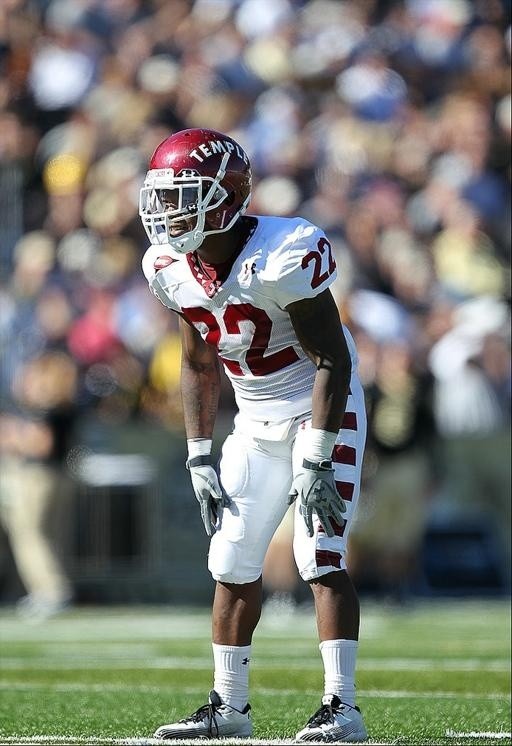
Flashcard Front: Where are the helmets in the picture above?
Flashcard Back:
[137,127,256,255]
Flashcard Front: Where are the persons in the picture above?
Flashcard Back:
[132,125,376,743]
[0,0,511,629]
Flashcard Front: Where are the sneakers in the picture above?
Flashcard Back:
[153,691,252,739]
[293,694,366,742]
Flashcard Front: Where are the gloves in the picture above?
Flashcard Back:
[184,451,231,537]
[286,455,347,539]
